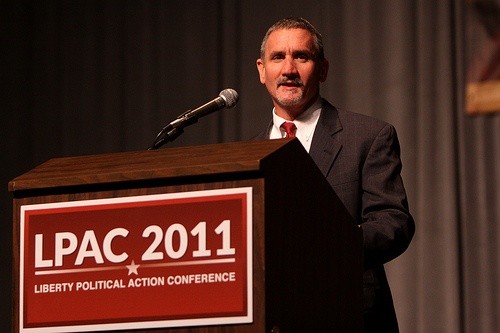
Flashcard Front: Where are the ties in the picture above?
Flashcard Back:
[280,121,297,137]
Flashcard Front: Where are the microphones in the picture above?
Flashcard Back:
[163,89,239,132]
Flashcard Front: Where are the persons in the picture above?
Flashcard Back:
[251,15,416,333]
